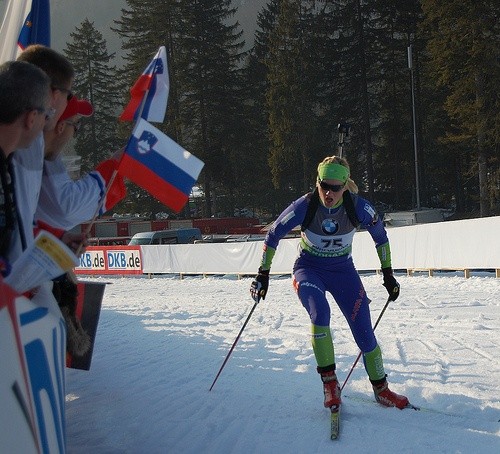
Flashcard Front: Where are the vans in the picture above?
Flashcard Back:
[84,236,132,246]
[127,227,203,246]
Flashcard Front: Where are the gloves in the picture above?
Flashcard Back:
[250,267,270,304]
[381,267,400,302]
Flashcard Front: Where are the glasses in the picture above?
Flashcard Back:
[65,118,83,130]
[57,88,74,101]
[316,176,348,193]
[33,105,56,120]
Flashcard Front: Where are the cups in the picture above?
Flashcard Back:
[61,155,81,182]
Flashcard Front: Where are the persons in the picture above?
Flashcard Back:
[9,44,76,266]
[0,61,52,274]
[36,96,127,256]
[251,156,409,408]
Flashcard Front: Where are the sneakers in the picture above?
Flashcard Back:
[371,374,413,410]
[319,368,341,413]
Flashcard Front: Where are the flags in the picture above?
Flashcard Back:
[120,46,170,123]
[119,120,205,213]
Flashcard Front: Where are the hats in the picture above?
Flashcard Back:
[59,95,94,125]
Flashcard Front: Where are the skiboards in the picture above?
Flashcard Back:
[344,395,500,422]
[329,404,340,439]
